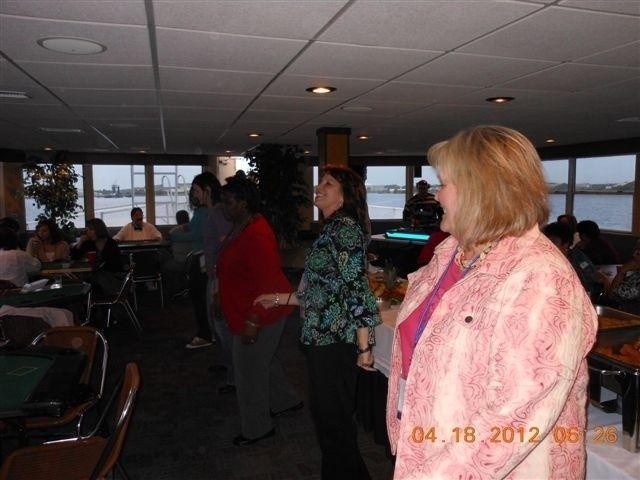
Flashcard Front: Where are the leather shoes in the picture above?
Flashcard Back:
[218,386,232,394]
[209,363,227,371]
[269,401,305,417]
[232,428,275,448]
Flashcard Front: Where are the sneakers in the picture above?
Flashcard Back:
[186,337,214,349]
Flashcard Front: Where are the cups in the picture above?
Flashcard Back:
[87,251,96,265]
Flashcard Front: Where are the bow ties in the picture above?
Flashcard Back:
[134,227,142,231]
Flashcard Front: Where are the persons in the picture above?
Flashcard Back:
[251,164,383,479]
[541,215,640,304]
[71,218,122,328]
[112,207,162,242]
[217,186,304,447]
[1,217,41,288]
[26,220,69,262]
[162,171,234,348]
[386,125,600,480]
[403,179,444,227]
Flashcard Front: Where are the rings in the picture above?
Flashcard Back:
[250,339,254,343]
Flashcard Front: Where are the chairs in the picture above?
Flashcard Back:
[0,361,142,480]
[0,236,198,440]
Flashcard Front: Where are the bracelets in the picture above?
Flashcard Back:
[286,292,292,307]
[245,321,259,328]
[358,347,371,353]
[274,292,279,308]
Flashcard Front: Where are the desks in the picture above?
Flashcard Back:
[586,385,640,480]
[370,268,412,377]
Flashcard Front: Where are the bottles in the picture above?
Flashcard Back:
[32,252,72,270]
